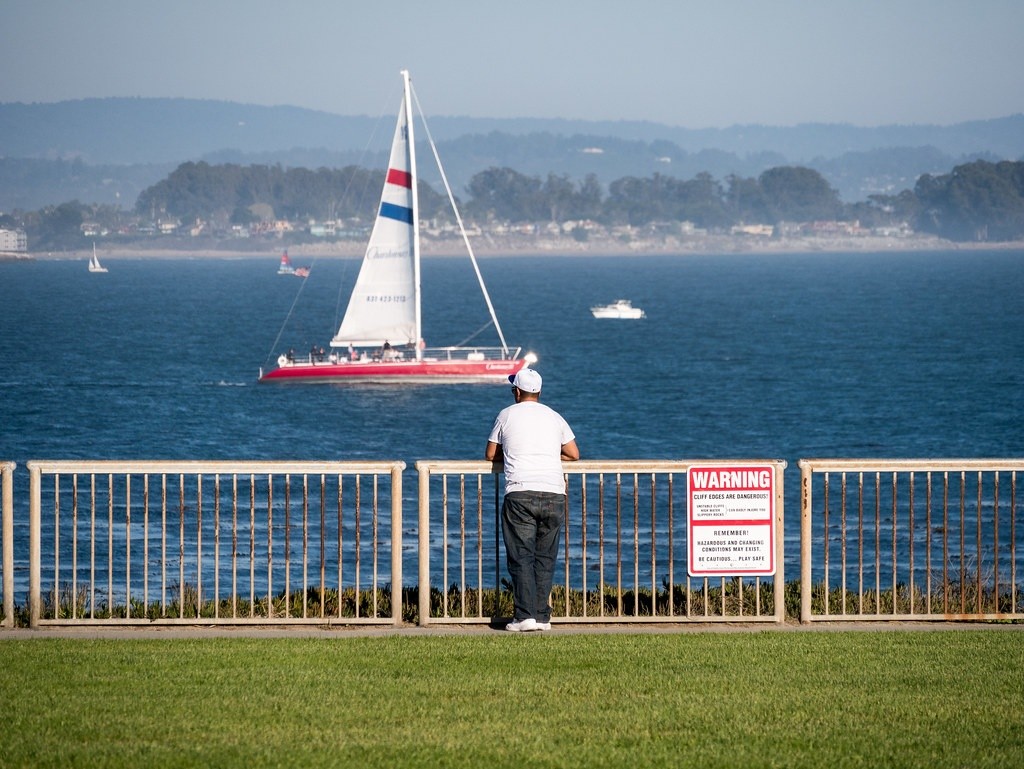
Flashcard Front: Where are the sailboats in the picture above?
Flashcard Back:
[88,241,111,273]
[258,68,537,384]
[276,248,296,274]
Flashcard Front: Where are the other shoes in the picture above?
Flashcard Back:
[536,622,551,630]
[505,618,536,631]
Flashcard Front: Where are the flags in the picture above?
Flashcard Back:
[293,265,310,277]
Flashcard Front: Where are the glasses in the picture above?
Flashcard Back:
[511,387,517,394]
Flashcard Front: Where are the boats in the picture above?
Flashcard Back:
[590,301,644,319]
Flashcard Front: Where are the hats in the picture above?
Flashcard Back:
[508,369,542,393]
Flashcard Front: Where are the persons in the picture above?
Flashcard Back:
[485,368,578,631]
[285,342,383,362]
[420,337,425,358]
[382,339,391,350]
